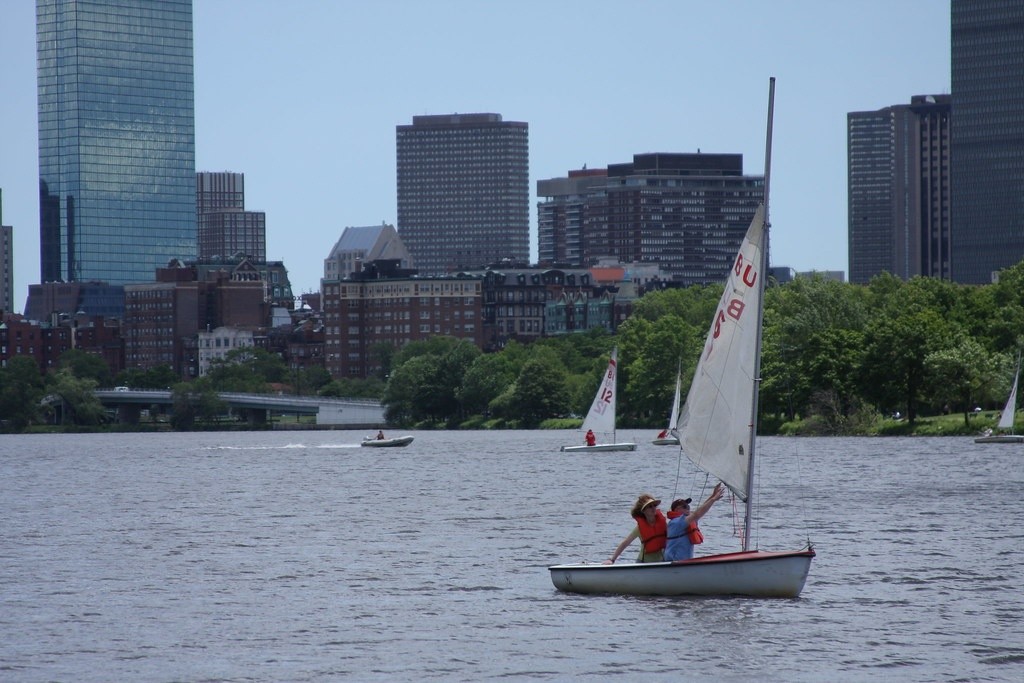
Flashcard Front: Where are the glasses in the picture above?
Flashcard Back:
[646,504,657,508]
[677,505,690,509]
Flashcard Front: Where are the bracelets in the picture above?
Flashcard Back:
[609,558,615,563]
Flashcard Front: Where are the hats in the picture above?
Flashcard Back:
[671,498,692,511]
[640,498,661,511]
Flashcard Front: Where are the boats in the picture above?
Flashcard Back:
[360,435,416,449]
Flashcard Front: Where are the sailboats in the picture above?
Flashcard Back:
[974,350,1024,443]
[652,348,681,447]
[559,343,639,454]
[547,72,815,601]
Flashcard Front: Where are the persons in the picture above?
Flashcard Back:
[600,481,723,565]
[377,430,384,440]
[663,485,727,562]
[585,430,596,446]
[657,429,670,439]
